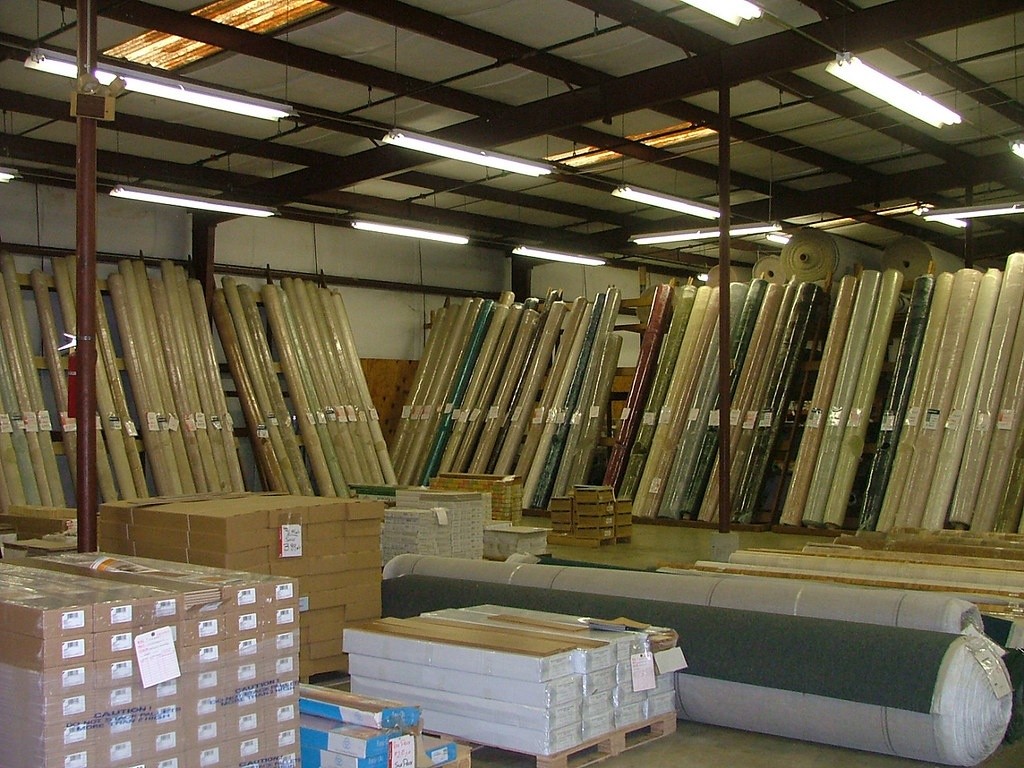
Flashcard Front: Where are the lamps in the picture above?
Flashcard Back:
[1,168,24,184]
[350,218,472,245]
[626,219,782,244]
[939,219,967,228]
[109,182,283,219]
[382,127,554,179]
[612,183,720,221]
[24,47,301,122]
[765,232,793,245]
[697,273,709,281]
[920,200,1024,221]
[680,0,766,27]
[512,245,607,267]
[1009,138,1024,160]
[825,52,963,130]
